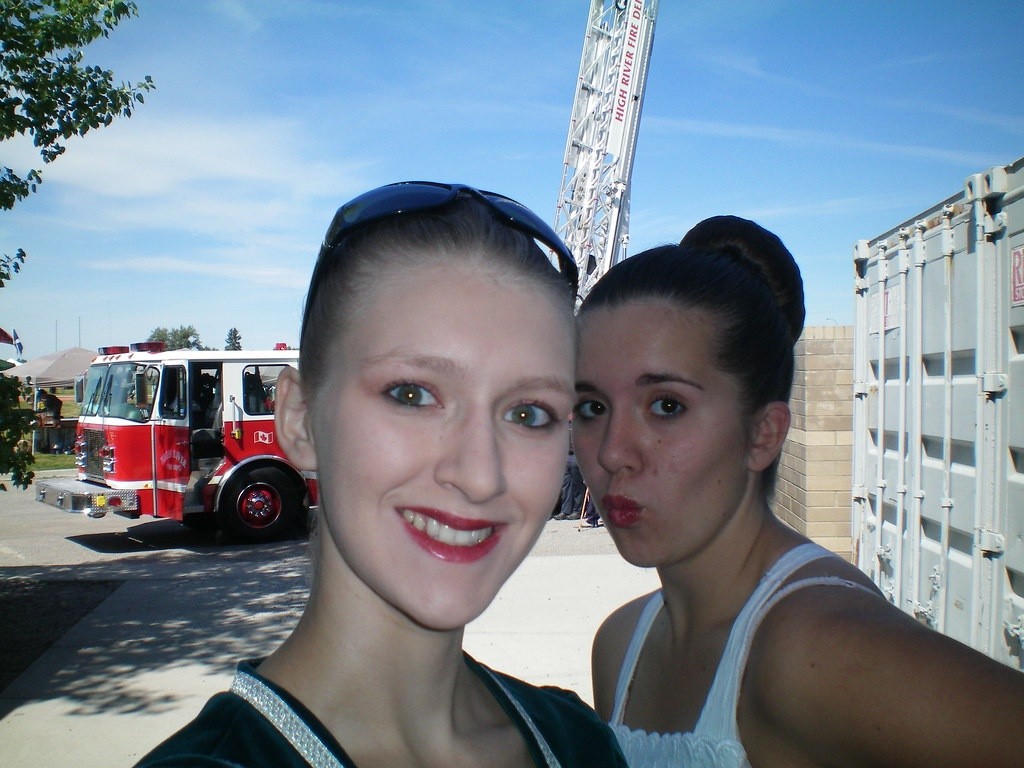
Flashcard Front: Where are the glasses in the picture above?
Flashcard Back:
[301,180,578,352]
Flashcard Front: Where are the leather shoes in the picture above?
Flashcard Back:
[566,513,581,520]
[554,513,567,519]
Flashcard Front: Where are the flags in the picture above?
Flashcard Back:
[13,328,23,355]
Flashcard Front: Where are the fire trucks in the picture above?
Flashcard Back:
[33,0,658,546]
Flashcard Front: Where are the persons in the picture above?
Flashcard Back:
[130,180,628,768]
[551,428,600,527]
[38,390,63,419]
[572,216,1024,768]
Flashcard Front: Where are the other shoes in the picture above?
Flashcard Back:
[573,520,598,528]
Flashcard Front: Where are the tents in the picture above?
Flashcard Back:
[0,347,101,456]
